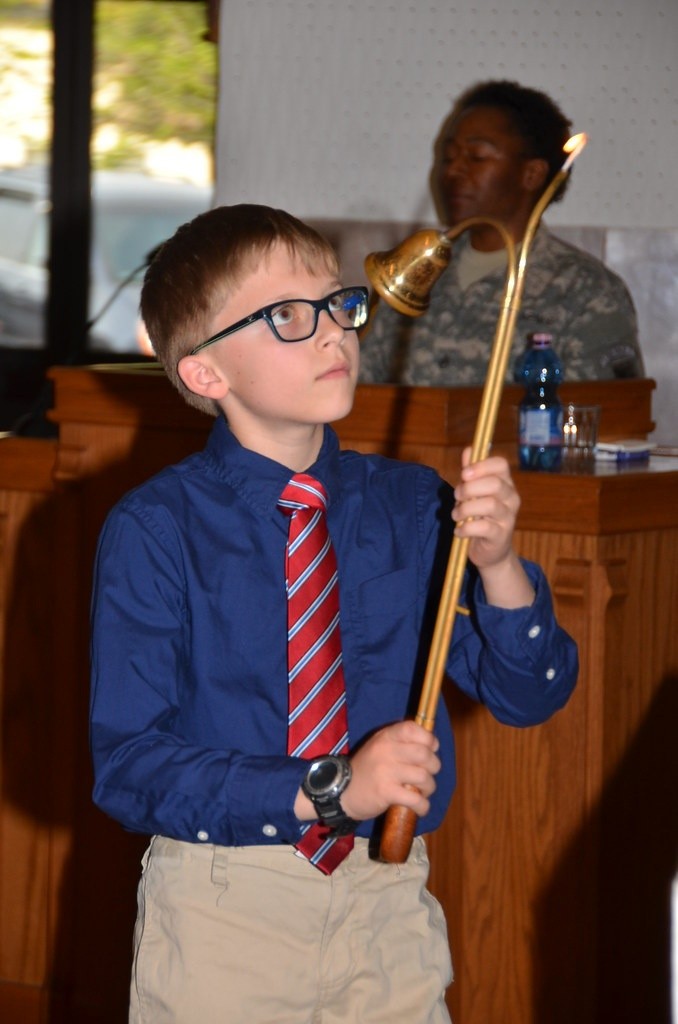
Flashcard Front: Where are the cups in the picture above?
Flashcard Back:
[561,403,599,457]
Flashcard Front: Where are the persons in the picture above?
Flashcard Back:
[358,78,642,386]
[85,202,581,1023]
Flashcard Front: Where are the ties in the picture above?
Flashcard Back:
[276,473,355,875]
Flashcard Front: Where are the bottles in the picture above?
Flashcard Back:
[515,333,564,469]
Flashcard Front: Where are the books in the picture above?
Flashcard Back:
[595,438,658,461]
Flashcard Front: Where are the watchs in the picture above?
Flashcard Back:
[301,753,362,835]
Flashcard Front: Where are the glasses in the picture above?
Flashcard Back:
[190,286,370,355]
